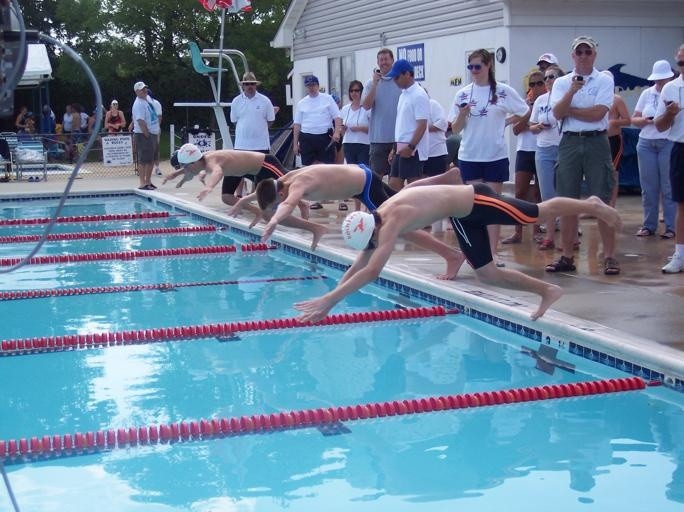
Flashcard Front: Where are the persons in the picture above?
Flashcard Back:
[579,71,631,219]
[293,183,624,324]
[424,88,454,230]
[360,48,404,183]
[447,49,531,267]
[131,82,160,190]
[231,72,276,197]
[128,89,162,175]
[15,99,126,166]
[228,164,466,281]
[161,150,254,216]
[445,122,462,168]
[384,59,432,232]
[166,143,309,229]
[293,75,348,210]
[322,95,344,204]
[341,80,369,212]
[653,44,684,274]
[501,52,582,250]
[631,60,676,239]
[659,68,680,221]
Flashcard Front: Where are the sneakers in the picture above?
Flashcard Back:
[662,252,684,273]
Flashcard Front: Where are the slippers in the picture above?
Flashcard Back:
[28,176,40,182]
[139,184,157,190]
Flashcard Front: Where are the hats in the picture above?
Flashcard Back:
[134,82,147,91]
[537,53,558,64]
[304,75,320,86]
[386,59,413,77]
[240,72,262,84]
[341,211,376,250]
[170,143,203,168]
[647,60,674,80]
[570,37,598,48]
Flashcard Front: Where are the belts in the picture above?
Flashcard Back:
[567,130,606,136]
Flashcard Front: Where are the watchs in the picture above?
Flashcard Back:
[408,144,415,150]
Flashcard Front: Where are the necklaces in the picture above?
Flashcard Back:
[469,80,491,116]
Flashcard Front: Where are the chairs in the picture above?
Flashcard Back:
[187,42,228,74]
[14,140,47,182]
[0,131,18,174]
[0,139,13,180]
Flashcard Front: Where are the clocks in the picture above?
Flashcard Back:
[495,47,506,64]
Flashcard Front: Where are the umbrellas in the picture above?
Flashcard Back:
[198,1,253,104]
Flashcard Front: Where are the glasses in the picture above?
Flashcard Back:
[467,65,481,70]
[350,89,360,93]
[677,60,683,67]
[529,80,543,87]
[544,74,555,81]
[366,242,374,250]
[575,49,592,56]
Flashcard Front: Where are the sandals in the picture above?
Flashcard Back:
[309,203,322,209]
[503,231,521,243]
[636,227,655,236]
[605,257,620,274]
[339,203,349,210]
[545,255,577,271]
[534,217,582,251]
[660,228,675,239]
